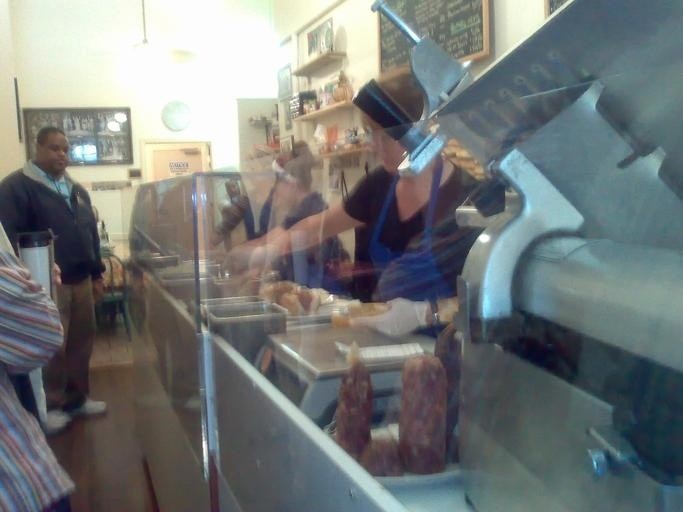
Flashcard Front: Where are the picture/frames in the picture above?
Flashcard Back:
[21,106,134,167]
[139,138,213,185]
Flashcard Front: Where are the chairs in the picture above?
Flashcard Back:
[91,253,131,346]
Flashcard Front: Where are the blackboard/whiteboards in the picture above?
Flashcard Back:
[543,0,569,19]
[377,0,490,79]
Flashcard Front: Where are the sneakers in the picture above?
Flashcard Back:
[74,399,108,419]
[39,409,71,435]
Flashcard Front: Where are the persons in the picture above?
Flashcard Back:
[0,220,77,511]
[204,145,275,251]
[203,72,505,342]
[0,125,106,438]
[255,138,352,297]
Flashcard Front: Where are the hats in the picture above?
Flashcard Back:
[272,140,315,188]
[352,69,431,152]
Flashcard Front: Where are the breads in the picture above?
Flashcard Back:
[335,355,447,476]
[331,303,389,327]
[264,280,312,316]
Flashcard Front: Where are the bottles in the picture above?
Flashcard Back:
[300,70,355,114]
[14,230,55,301]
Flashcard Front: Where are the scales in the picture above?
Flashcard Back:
[255,324,429,426]
